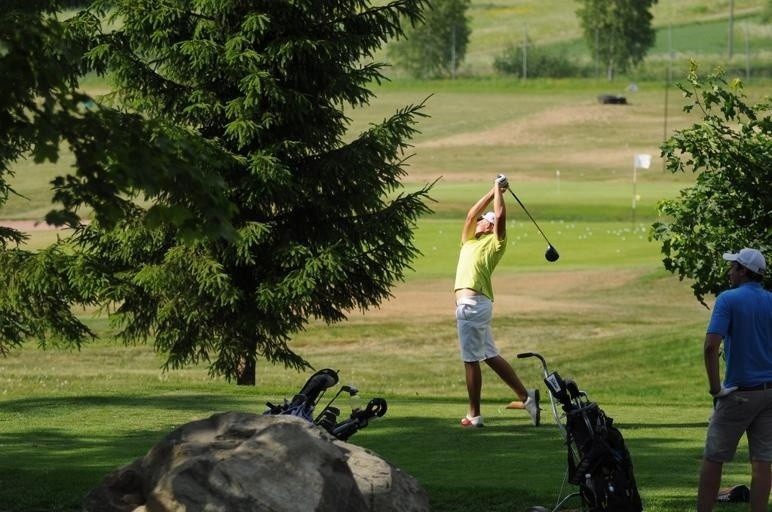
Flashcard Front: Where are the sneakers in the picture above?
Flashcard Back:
[460,410,484,427]
[522,388,540,427]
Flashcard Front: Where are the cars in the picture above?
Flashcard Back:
[596,93,627,104]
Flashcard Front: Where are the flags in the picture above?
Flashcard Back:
[634,153,653,170]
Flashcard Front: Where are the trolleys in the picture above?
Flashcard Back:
[514,353,644,512]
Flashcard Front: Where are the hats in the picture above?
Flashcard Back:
[723,247,766,275]
[480,211,495,225]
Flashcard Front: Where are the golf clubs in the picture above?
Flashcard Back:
[544,371,590,412]
[262,369,388,443]
[497,174,559,262]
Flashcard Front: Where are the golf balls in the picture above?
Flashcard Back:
[509,218,651,247]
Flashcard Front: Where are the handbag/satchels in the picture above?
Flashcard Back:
[564,400,643,510]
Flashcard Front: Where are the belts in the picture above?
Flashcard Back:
[737,382,772,391]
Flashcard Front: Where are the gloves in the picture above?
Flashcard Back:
[495,173,508,188]
[708,381,739,399]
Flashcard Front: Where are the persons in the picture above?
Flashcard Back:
[695,247,772,511]
[454,173,541,429]
